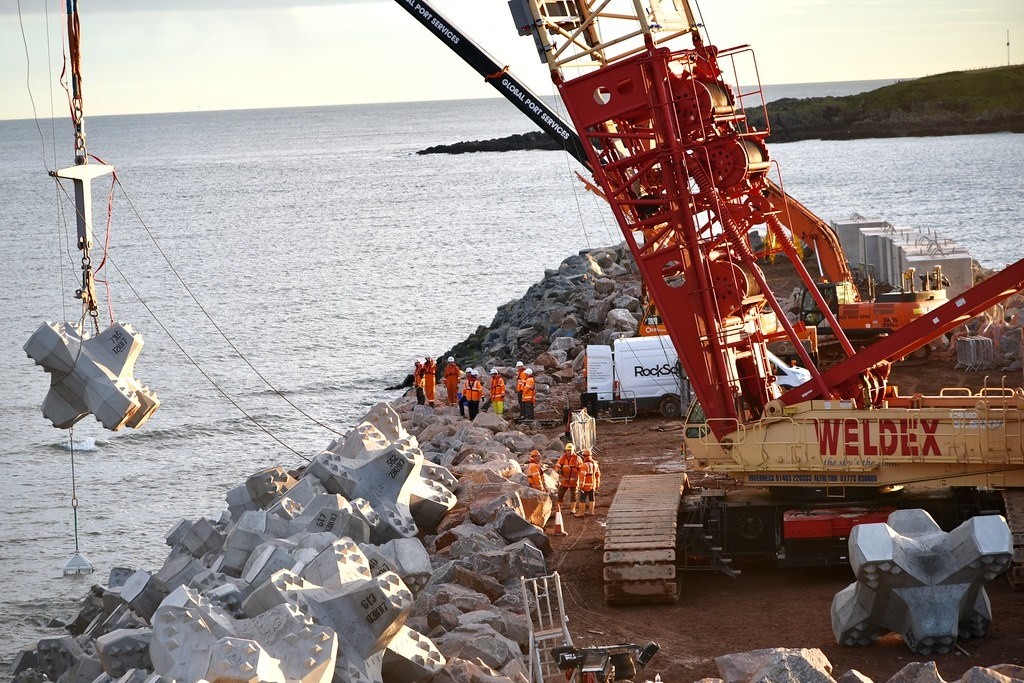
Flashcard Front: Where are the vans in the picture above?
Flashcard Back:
[586,334,813,418]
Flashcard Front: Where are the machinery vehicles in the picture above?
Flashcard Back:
[614,72,955,361]
[42,0,1024,609]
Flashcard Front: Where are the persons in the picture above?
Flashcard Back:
[490,369,505,417]
[414,358,425,405]
[421,354,436,408]
[527,450,545,491]
[462,370,484,421]
[516,361,527,420]
[460,368,473,417]
[575,450,601,517]
[521,368,535,420]
[443,356,461,405]
[554,443,583,514]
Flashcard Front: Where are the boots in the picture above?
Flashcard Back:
[574,502,585,517]
[570,502,576,513]
[585,501,595,515]
[557,502,562,512]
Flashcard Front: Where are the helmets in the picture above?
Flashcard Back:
[489,369,498,374]
[516,361,524,366]
[565,443,574,450]
[448,356,455,362]
[471,370,480,375]
[524,368,533,374]
[530,450,541,456]
[465,367,472,373]
[414,358,420,363]
[582,450,592,456]
